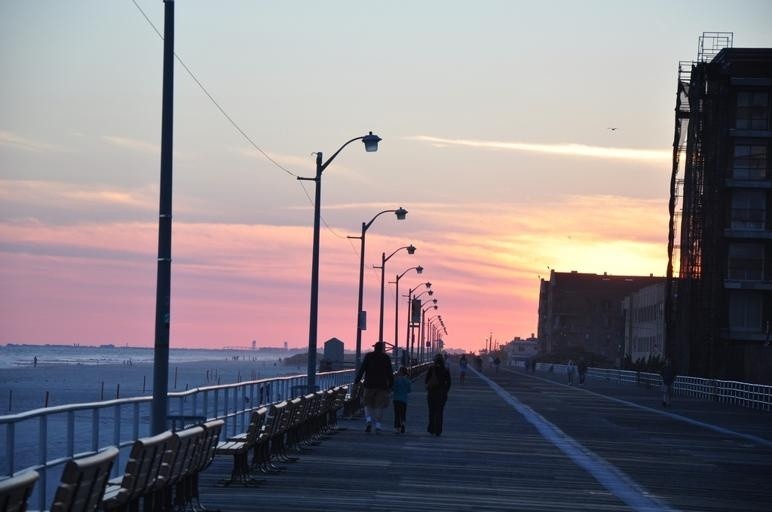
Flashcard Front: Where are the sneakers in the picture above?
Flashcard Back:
[365,422,405,435]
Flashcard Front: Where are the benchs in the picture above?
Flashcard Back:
[340,362,432,421]
[213,385,349,489]
[0,419,226,511]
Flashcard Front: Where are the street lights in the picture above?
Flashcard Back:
[345,203,412,369]
[403,280,449,366]
[294,127,382,401]
[387,263,425,370]
[373,242,418,343]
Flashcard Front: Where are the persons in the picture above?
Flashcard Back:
[391,367,412,434]
[459,356,467,385]
[567,360,575,386]
[662,361,672,408]
[474,353,501,373]
[424,354,451,437]
[443,353,450,368]
[352,341,392,433]
[577,356,586,386]
[524,359,528,372]
[532,359,536,372]
